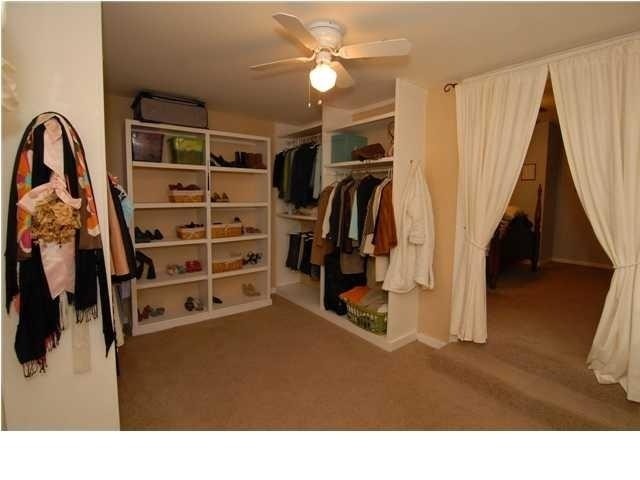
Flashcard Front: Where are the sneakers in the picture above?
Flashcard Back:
[168,261,203,275]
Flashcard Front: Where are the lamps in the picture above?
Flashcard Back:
[309,52,336,91]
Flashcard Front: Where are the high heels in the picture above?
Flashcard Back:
[213,192,221,202]
[185,297,206,312]
[241,250,264,264]
[222,192,229,202]
[242,283,260,297]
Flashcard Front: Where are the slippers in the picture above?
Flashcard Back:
[247,226,261,233]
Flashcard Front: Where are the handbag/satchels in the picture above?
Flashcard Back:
[352,144,385,160]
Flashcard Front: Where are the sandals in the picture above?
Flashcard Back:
[138,305,164,322]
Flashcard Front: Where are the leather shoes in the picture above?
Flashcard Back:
[135,227,162,242]
[213,296,222,303]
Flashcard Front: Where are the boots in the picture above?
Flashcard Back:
[135,251,154,279]
[210,152,266,168]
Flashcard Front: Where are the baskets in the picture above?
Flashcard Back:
[213,260,242,273]
[346,299,386,336]
[211,224,242,238]
[176,226,206,240]
[168,189,203,202]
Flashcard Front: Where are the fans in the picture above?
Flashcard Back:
[249,13,410,91]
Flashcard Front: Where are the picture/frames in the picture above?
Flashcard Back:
[521,164,536,180]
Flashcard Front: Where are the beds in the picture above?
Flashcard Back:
[484,206,545,288]
